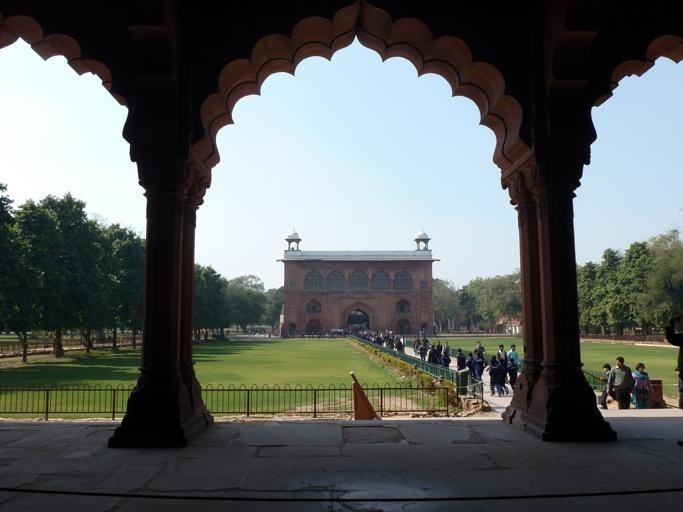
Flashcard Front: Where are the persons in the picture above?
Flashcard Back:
[664,313,682,448]
[598,363,617,409]
[343,325,519,398]
[628,362,654,409]
[606,356,634,409]
